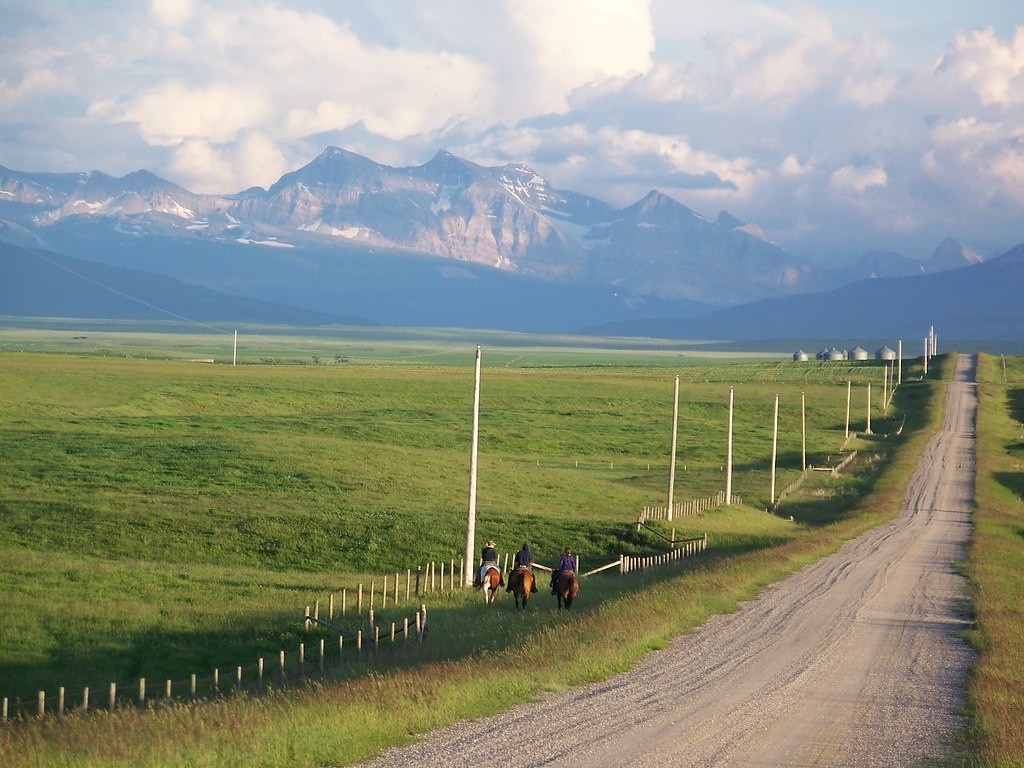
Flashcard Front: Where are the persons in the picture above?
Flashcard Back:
[473,540,505,588]
[505,544,538,594]
[551,546,575,596]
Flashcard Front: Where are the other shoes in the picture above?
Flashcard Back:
[551,591,556,595]
[532,589,537,593]
[506,589,510,593]
[500,584,504,586]
[473,583,478,587]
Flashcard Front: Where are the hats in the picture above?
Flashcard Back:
[486,541,494,548]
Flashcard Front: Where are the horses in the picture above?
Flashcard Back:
[510,560,533,610]
[482,567,501,606]
[549,568,579,613]
